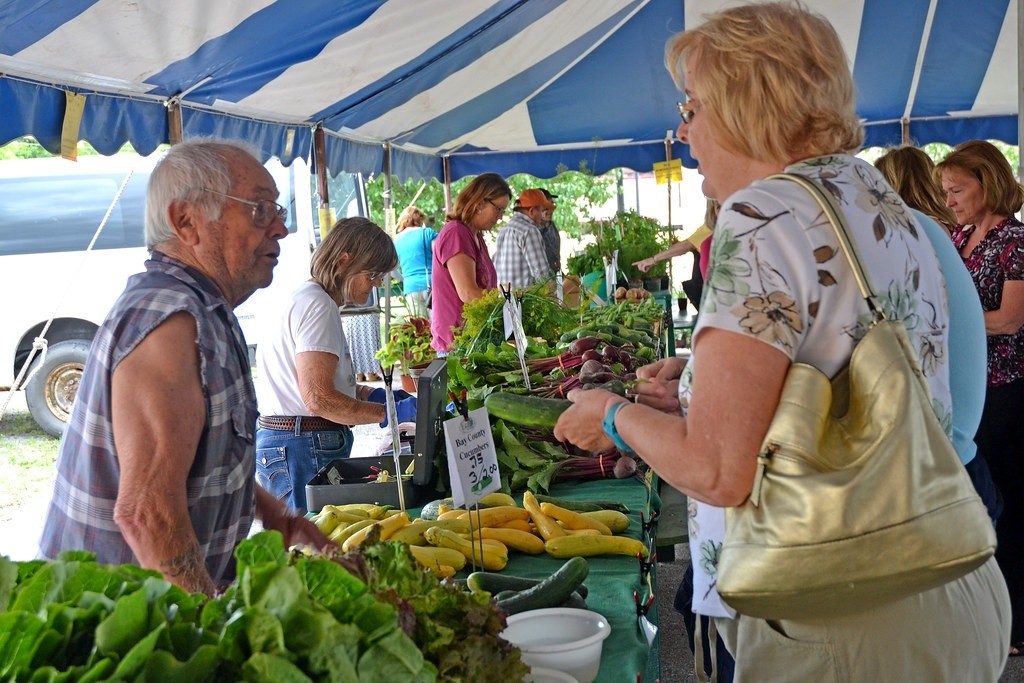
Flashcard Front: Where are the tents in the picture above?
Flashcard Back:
[0,0,1024,342]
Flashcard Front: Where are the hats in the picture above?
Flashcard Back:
[516,189,556,210]
[538,187,558,200]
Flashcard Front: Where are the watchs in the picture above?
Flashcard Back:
[602,400,633,453]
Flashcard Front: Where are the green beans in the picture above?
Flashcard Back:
[583,298,667,327]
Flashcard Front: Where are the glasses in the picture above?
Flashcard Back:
[190,186,287,227]
[677,100,700,124]
[365,270,386,281]
[487,198,506,215]
[550,201,557,207]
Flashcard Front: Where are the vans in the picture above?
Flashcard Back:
[0,148,381,439]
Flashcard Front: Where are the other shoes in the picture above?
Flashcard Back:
[1008,643,1024,657]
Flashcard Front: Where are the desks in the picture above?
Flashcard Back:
[437,344,662,683]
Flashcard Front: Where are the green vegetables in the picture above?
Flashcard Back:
[0,522,534,683]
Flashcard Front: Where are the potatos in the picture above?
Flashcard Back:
[612,287,652,301]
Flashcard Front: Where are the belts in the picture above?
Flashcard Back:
[257,414,353,432]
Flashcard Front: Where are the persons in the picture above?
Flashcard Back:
[344,280,382,382]
[254,217,417,514]
[34,140,332,601]
[493,188,560,295]
[431,171,512,358]
[633,196,722,310]
[869,140,1024,656]
[554,0,1011,683]
[392,206,440,321]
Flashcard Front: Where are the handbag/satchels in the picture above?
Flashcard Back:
[713,174,999,621]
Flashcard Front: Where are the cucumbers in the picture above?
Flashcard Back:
[452,555,590,613]
[483,379,628,428]
[558,321,657,362]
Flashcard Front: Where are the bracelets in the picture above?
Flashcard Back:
[652,255,657,266]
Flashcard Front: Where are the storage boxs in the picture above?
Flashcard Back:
[306,454,415,514]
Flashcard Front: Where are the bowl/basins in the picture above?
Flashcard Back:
[496,607,611,683]
